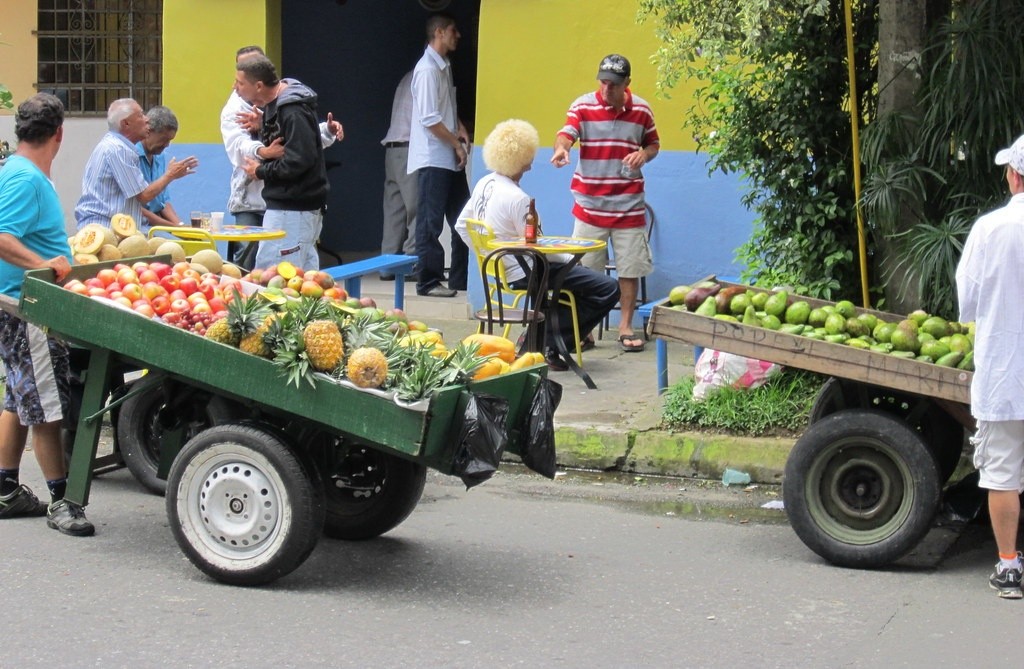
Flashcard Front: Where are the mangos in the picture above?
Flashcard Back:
[243,262,428,338]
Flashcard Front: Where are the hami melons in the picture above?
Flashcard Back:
[68,213,242,278]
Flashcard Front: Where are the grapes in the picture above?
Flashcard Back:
[169,308,220,335]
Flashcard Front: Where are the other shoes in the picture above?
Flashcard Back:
[380,273,418,281]
[543,347,569,370]
[425,283,458,296]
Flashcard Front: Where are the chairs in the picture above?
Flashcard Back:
[474,247,550,359]
[597,200,655,340]
[466,217,583,367]
[148,225,217,257]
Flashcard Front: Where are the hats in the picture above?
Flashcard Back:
[596,53,631,83]
[995,133,1024,175]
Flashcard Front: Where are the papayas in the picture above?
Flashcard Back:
[399,329,547,380]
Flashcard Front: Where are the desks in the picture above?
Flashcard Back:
[486,236,607,368]
[172,225,285,241]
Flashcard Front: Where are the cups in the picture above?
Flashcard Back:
[211,212,224,233]
[722,469,751,486]
[200,213,212,231]
[190,211,201,229]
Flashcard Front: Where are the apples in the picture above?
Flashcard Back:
[64,261,246,326]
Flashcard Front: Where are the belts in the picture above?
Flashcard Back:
[386,140,409,147]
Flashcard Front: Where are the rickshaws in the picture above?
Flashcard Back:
[0,254,549,587]
[644,275,977,569]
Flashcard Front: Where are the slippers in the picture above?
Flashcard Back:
[617,332,644,351]
[571,335,595,353]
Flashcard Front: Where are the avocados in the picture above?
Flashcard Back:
[665,281,974,373]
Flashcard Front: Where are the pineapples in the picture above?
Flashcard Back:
[204,285,390,389]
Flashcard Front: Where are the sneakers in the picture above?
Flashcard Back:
[989,560,1023,598]
[47,499,96,536]
[0,483,50,518]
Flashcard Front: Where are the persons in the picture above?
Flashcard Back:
[232,55,329,274]
[550,55,659,351]
[454,120,622,371]
[380,69,419,281]
[74,99,199,232]
[408,15,471,296]
[134,105,190,227]
[0,93,95,536]
[955,134,1024,598]
[220,45,345,276]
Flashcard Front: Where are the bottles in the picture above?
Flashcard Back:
[525,198,538,244]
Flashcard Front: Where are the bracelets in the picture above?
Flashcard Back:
[177,221,185,226]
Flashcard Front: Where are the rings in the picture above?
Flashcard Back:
[187,167,190,172]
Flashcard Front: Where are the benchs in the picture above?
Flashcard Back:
[321,254,419,311]
[638,276,757,399]
